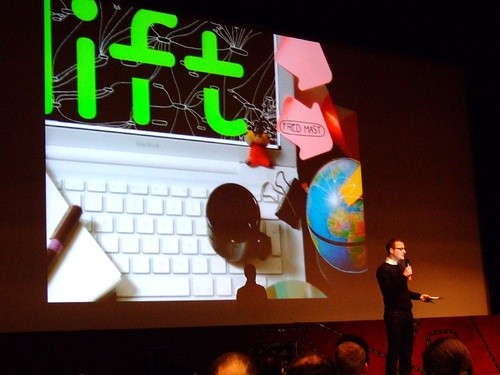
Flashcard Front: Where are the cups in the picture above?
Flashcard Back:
[206,183,270,262]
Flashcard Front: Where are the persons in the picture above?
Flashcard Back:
[422,339,472,375]
[375,239,433,375]
[211,340,370,375]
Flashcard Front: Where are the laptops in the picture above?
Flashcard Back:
[46,0,306,302]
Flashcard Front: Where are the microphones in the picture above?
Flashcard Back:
[405,259,412,280]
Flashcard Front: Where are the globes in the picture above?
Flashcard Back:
[306,157,368,274]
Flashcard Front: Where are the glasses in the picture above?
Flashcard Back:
[392,247,406,250]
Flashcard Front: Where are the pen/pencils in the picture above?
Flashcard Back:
[46,205,82,277]
